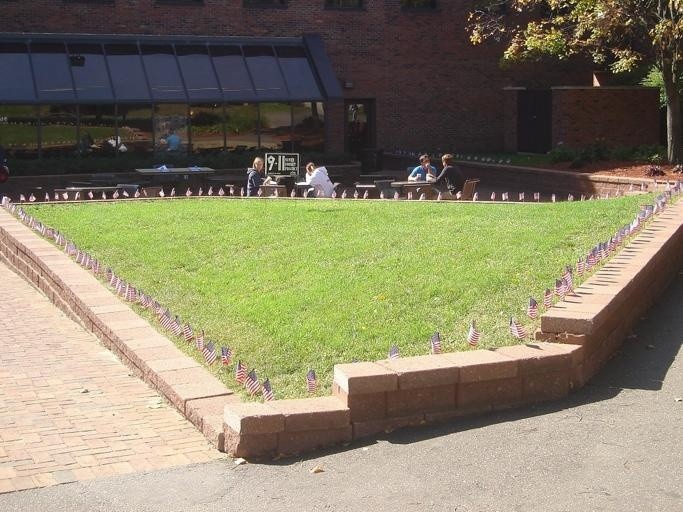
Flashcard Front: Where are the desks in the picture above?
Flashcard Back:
[136,167,215,194]
[391,181,434,200]
[258,181,310,196]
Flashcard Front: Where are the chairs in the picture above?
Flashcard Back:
[405,184,434,200]
[441,178,479,199]
[329,182,343,198]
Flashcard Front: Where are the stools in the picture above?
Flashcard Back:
[355,184,376,198]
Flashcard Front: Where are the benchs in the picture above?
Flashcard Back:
[54,184,138,200]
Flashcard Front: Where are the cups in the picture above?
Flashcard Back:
[416,174,422,182]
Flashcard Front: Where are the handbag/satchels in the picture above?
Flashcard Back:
[277,176,296,185]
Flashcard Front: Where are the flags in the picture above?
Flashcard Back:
[431,332,441,354]
[380,181,659,203]
[20,188,147,204]
[389,346,399,359]
[656,179,683,205]
[468,319,480,345]
[158,188,370,199]
[2,197,274,403]
[528,202,661,318]
[510,317,528,339]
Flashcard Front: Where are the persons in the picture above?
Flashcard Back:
[305,162,334,198]
[407,154,437,200]
[164,129,181,151]
[246,156,272,196]
[426,153,464,199]
